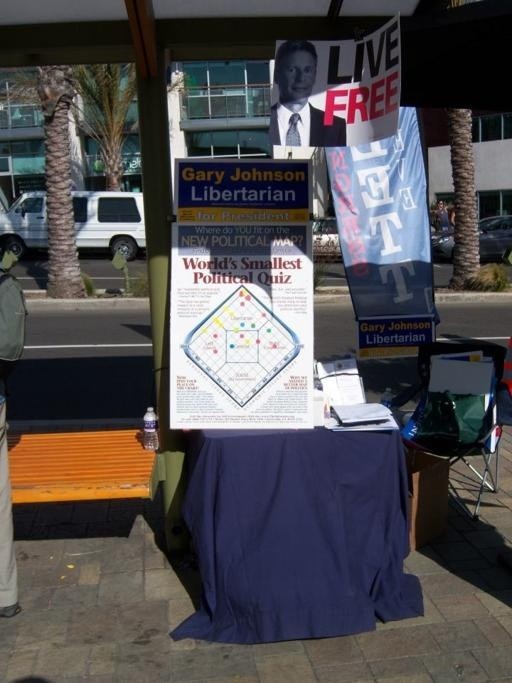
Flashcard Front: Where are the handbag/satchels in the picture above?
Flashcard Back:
[0,270,26,362]
[412,391,485,452]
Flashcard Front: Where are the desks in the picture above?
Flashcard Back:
[168,401,423,644]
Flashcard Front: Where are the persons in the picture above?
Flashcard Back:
[449,204,455,230]
[270,36,345,147]
[433,199,451,230]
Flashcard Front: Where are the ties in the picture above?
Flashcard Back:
[286,114,302,145]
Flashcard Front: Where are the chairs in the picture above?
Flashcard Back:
[387,341,508,530]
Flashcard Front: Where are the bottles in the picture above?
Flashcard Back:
[142,406,159,451]
[380,387,393,408]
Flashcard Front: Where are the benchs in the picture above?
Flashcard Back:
[7,430,160,506]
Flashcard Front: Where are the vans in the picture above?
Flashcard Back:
[0,190,147,262]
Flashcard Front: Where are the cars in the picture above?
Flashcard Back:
[431,214,512,266]
[310,216,343,258]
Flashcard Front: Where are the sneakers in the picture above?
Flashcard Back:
[0,603,18,617]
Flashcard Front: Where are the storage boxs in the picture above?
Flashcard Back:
[407,452,449,551]
